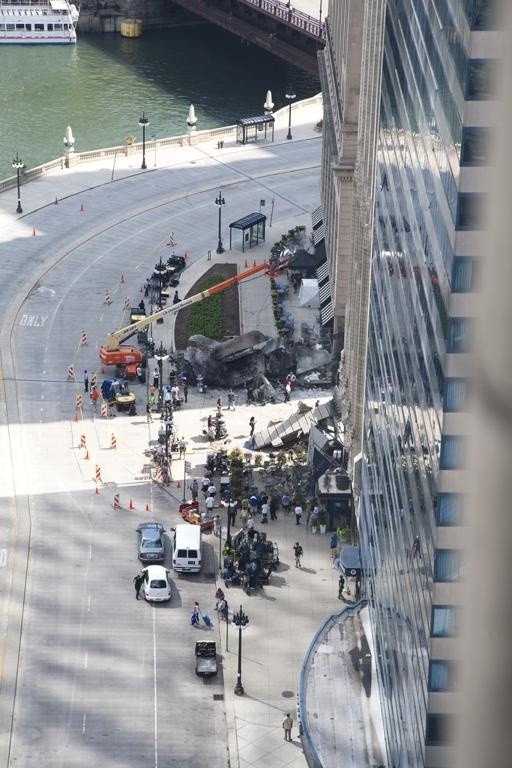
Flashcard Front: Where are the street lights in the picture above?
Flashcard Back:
[153,340,171,412]
[228,602,251,697]
[119,273,126,283]
[157,422,177,463]
[11,151,25,214]
[80,204,84,211]
[32,227,36,237]
[285,84,297,140]
[137,110,150,169]
[153,254,167,326]
[219,486,239,550]
[213,188,226,254]
[54,196,58,204]
[221,607,230,652]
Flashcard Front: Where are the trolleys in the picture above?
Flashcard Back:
[246,383,275,405]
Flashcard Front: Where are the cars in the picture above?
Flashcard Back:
[140,562,172,603]
[145,271,169,288]
[164,254,186,273]
[133,521,168,564]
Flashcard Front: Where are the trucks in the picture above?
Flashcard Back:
[194,639,218,674]
[168,522,204,574]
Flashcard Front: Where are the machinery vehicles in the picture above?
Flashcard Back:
[114,379,136,410]
[97,242,321,381]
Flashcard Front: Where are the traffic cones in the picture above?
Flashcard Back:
[84,449,91,460]
[94,486,100,495]
[183,250,188,258]
[128,498,135,510]
[244,258,249,268]
[252,258,257,267]
[145,502,150,511]
[176,481,181,488]
[74,414,79,422]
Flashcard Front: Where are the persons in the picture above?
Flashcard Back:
[282,712,294,741]
[133,574,143,600]
[214,588,228,620]
[413,535,422,559]
[338,575,346,600]
[191,602,200,625]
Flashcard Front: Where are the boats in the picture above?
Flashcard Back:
[0,0,82,48]
